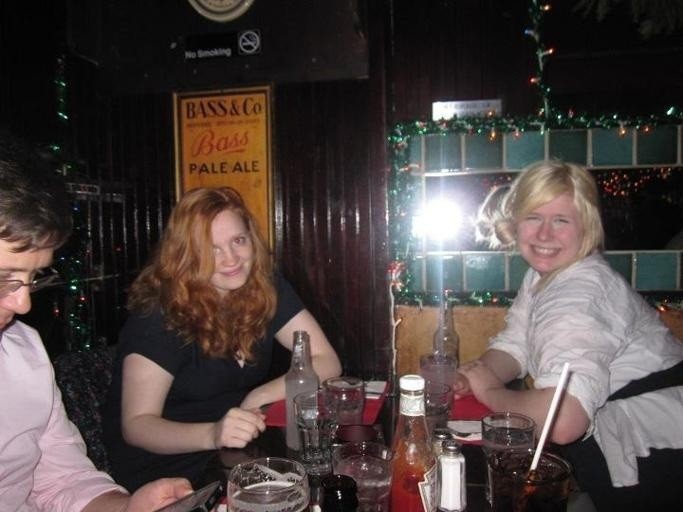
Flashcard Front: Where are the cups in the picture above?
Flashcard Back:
[420,355,467,411]
[295,375,392,512]
[227,456,310,511]
[481,411,571,512]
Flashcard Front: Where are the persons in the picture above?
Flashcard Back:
[451,157,683,512]
[116,186,344,493]
[1,139,197,511]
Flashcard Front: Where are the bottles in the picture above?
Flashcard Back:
[283,330,319,450]
[434,290,460,357]
[394,373,468,512]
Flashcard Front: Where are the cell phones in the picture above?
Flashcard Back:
[151,481,227,512]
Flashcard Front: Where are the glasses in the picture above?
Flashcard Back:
[0,267,59,298]
